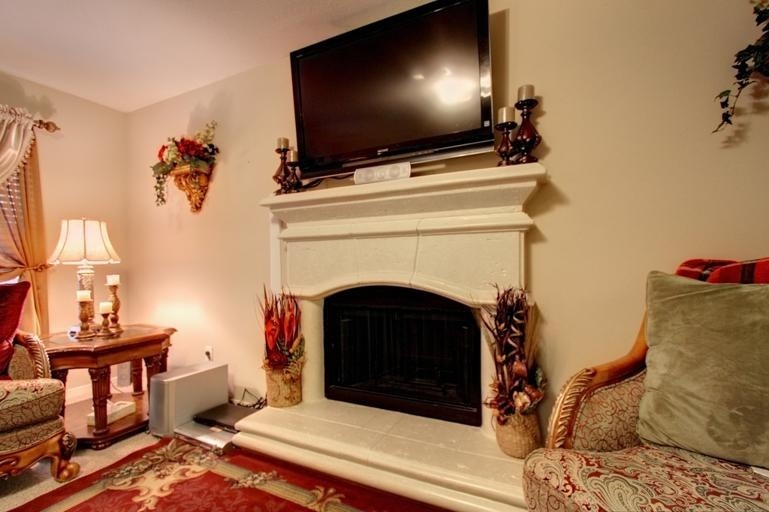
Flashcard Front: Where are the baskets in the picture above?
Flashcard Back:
[496,412,541,459]
[265,368,301,407]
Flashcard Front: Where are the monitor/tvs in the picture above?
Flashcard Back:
[289,0,498,180]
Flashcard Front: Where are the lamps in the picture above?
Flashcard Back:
[46,213,121,332]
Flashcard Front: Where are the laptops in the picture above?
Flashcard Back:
[193,403,259,434]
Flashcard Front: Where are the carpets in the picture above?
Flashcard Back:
[6,433,461,512]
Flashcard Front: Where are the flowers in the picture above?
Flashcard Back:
[146,119,220,210]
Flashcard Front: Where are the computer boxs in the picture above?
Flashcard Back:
[148,360,229,438]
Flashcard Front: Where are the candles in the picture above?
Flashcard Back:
[105,273,122,285]
[287,149,298,163]
[276,136,290,149]
[516,84,536,101]
[496,105,516,126]
[75,289,95,302]
[97,300,114,314]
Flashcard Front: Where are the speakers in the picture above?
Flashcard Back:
[353,161,413,184]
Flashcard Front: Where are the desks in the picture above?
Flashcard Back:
[32,320,178,457]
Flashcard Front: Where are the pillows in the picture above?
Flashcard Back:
[0,279,34,376]
[631,268,767,475]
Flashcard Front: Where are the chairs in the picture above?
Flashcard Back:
[0,324,83,484]
[517,254,768,510]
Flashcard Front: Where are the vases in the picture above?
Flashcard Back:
[489,399,546,458]
[168,159,212,214]
[259,357,304,411]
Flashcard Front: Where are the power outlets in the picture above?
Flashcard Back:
[204,344,214,363]
